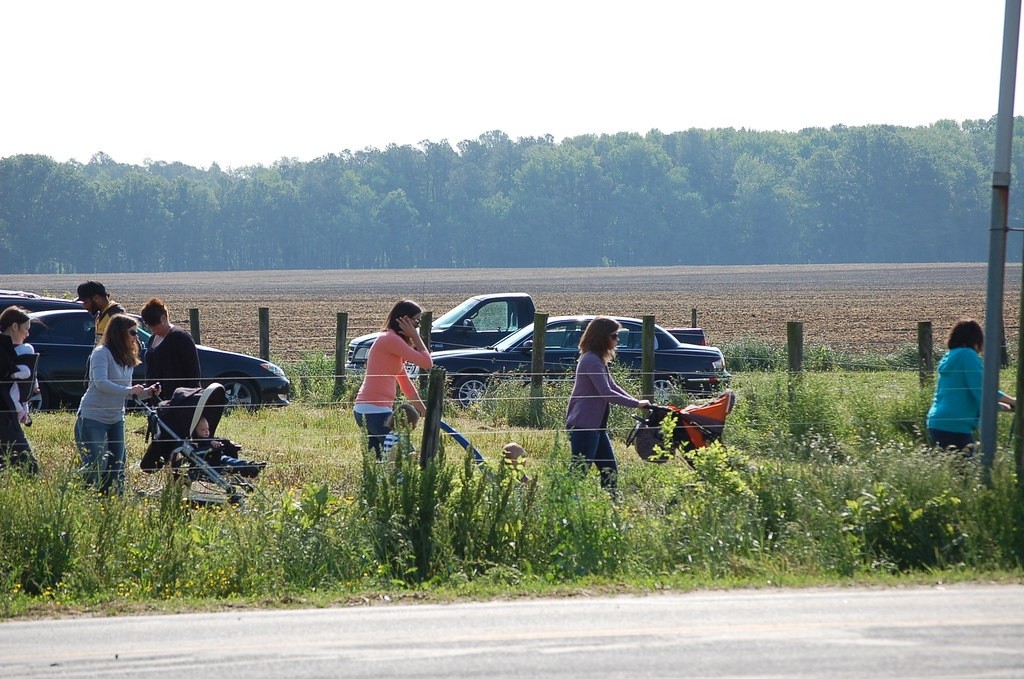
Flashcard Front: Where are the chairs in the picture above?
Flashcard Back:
[506,311,519,331]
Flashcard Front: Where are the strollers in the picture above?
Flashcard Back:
[625,387,735,468]
[128,382,254,511]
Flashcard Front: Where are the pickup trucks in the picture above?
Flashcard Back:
[344,294,705,410]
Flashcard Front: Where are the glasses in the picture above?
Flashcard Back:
[410,317,421,325]
[129,330,139,336]
[609,333,619,340]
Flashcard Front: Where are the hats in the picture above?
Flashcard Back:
[75,280,106,301]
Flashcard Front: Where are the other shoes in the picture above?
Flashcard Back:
[240,460,267,479]
[18,411,28,425]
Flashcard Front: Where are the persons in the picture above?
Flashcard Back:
[353,298,434,491]
[76,313,162,503]
[191,417,268,470]
[925,317,1018,460]
[498,441,544,495]
[142,299,200,401]
[78,281,130,364]
[565,311,654,502]
[671,399,720,419]
[2,304,45,485]
[382,402,421,488]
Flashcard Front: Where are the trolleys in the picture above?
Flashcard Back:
[440,423,539,499]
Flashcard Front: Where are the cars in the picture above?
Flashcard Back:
[0,290,291,413]
[405,317,731,399]
